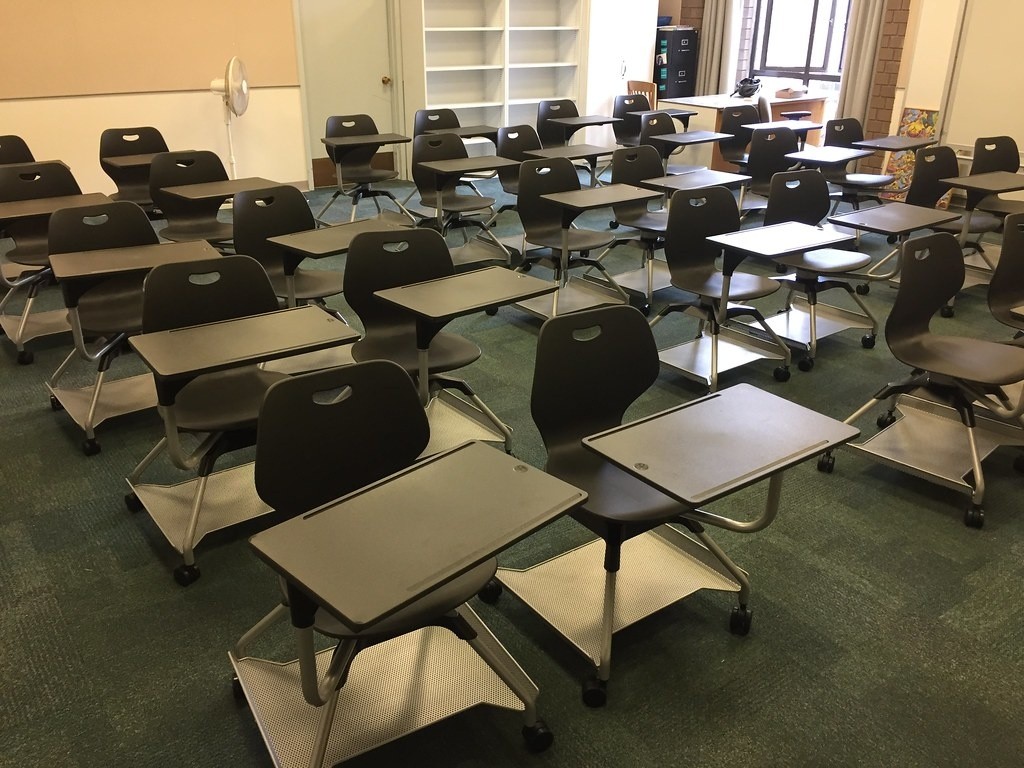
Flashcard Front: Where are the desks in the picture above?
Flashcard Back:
[247,438,247,438]
[522,143,614,160]
[0,192,114,220]
[159,176,282,201]
[659,91,828,173]
[248,438,588,631]
[828,201,963,236]
[581,381,862,506]
[649,130,735,144]
[321,132,412,149]
[128,304,361,378]
[937,170,1024,194]
[852,135,938,152]
[424,125,498,140]
[706,220,856,258]
[266,218,413,259]
[639,167,753,194]
[627,108,699,118]
[740,120,823,131]
[784,145,875,164]
[373,265,562,322]
[48,239,224,281]
[546,115,624,127]
[0,160,71,170]
[102,150,196,168]
[538,183,666,211]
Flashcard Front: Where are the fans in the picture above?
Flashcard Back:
[209,55,249,199]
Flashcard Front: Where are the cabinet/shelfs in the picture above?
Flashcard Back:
[653,30,699,98]
[398,0,582,181]
[569,0,658,170]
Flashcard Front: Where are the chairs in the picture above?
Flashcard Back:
[0,94,1024,767]
[627,80,657,123]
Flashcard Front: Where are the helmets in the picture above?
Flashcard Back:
[738,78,761,97]
